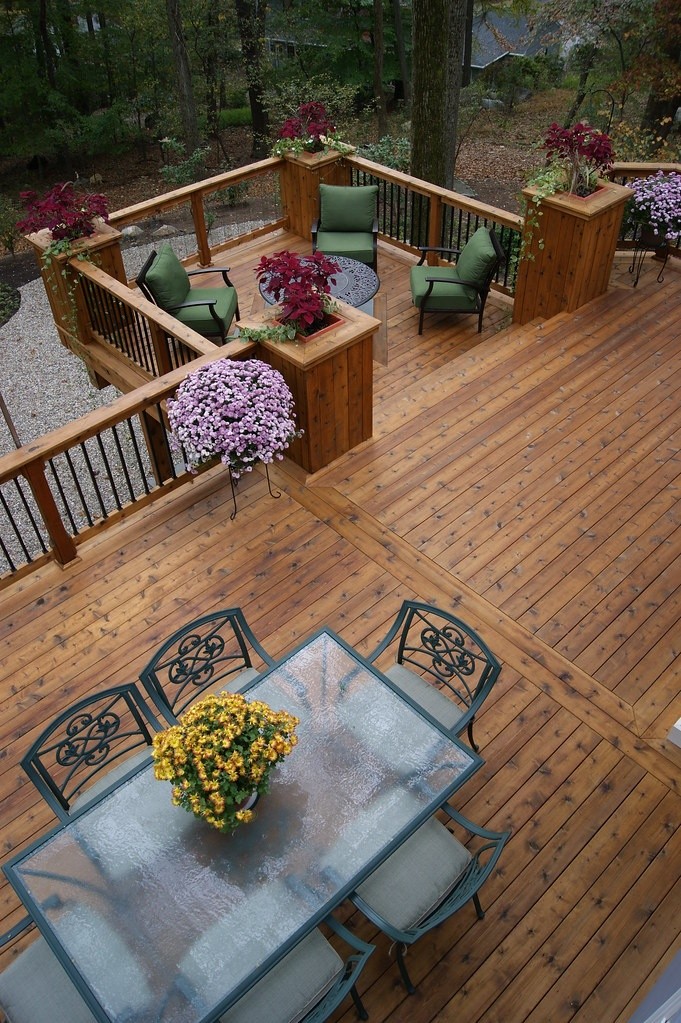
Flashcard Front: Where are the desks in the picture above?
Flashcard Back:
[259,254,380,318]
[0,624,486,1023]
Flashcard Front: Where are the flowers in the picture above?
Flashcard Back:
[165,358,307,476]
[621,167,681,247]
[149,691,299,834]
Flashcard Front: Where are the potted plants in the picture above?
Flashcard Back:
[259,102,350,157]
[9,182,119,246]
[252,247,346,344]
[534,118,610,201]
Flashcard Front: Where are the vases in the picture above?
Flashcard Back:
[641,218,667,248]
[232,787,260,814]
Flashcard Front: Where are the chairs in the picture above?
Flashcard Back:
[310,183,380,274]
[0,598,514,1023]
[409,226,506,333]
[134,242,241,349]
